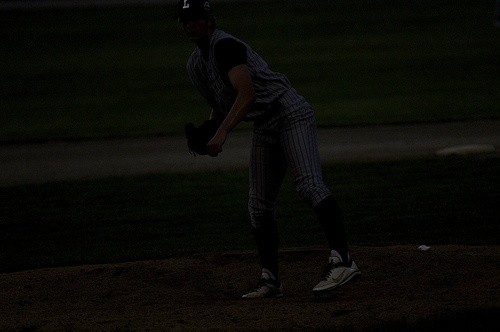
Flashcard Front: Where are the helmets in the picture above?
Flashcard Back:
[171,0,215,21]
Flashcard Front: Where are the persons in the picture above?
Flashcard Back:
[173,1,361,299]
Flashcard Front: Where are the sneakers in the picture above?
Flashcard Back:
[241,268,284,300]
[311,250,362,296]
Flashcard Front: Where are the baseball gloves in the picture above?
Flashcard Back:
[186,116,225,157]
